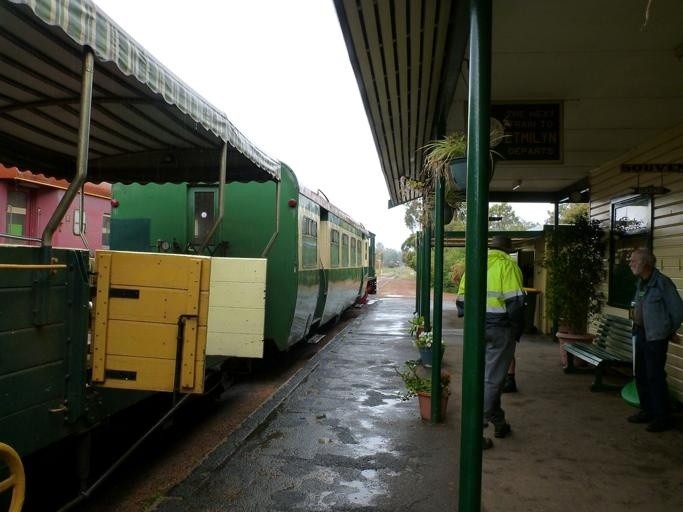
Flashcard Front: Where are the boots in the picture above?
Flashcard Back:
[502,372,518,393]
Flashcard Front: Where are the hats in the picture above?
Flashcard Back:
[486,235,517,253]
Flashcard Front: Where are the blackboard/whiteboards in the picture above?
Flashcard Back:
[490,99,565,165]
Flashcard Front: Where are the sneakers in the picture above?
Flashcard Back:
[481,437,492,450]
[494,423,510,438]
[627,409,675,432]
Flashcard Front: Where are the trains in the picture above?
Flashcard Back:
[0,0,379,512]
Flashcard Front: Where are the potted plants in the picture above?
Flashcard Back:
[535,209,614,369]
[407,312,432,339]
[396,359,451,423]
[419,131,512,193]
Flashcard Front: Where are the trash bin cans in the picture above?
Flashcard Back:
[523,288,541,334]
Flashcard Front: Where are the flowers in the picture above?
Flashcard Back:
[415,331,444,350]
[398,174,433,233]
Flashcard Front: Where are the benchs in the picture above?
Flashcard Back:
[562,313,633,392]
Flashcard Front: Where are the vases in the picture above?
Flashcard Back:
[420,348,444,365]
[429,201,454,225]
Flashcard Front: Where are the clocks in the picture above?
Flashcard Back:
[490,115,505,148]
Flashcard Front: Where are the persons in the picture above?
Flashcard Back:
[500,285,528,393]
[456,236,530,448]
[625,249,682,432]
